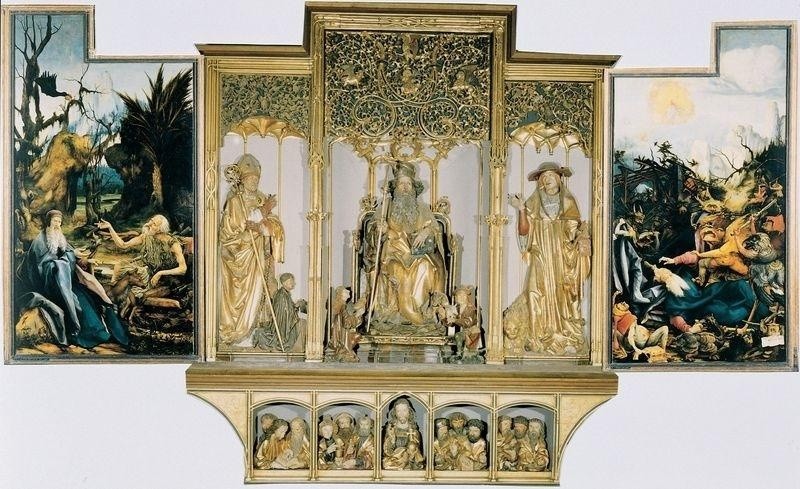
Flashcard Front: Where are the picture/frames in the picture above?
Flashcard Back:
[607,23,795,374]
[4,5,198,365]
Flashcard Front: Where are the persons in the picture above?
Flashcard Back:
[501,307,528,357]
[217,154,285,346]
[362,159,448,335]
[252,397,551,472]
[251,272,309,351]
[94,213,189,325]
[502,161,593,357]
[324,285,367,362]
[443,285,486,358]
[13,209,133,348]
[611,182,784,362]
